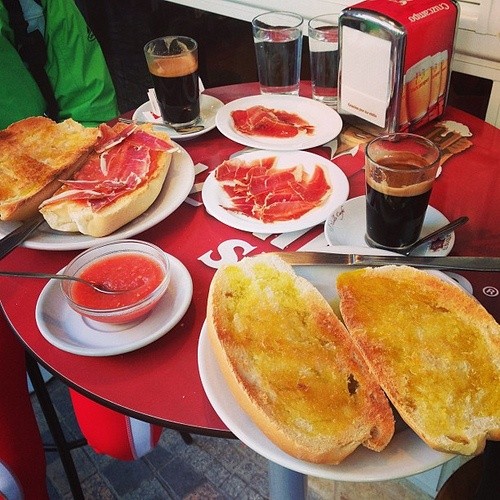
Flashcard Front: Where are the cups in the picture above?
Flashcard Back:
[143,37,200,126]
[365,133,440,249]
[251,10,304,97]
[308,13,341,110]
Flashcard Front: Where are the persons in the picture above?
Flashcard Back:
[0,0,164,500]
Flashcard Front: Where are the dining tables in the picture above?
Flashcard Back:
[0,80,500,500]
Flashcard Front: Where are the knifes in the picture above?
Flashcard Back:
[250,252,500,271]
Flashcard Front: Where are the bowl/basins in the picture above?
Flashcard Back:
[61,239,173,325]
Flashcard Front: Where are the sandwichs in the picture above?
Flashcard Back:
[0,116,180,239]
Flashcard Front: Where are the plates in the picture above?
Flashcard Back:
[35,252,193,358]
[132,95,225,142]
[0,129,196,251]
[197,261,456,482]
[202,150,349,232]
[325,195,456,256]
[215,94,344,151]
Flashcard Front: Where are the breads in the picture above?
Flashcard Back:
[205,251,500,466]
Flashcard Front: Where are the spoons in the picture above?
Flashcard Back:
[118,117,205,134]
[388,214,467,256]
[1,269,149,294]
